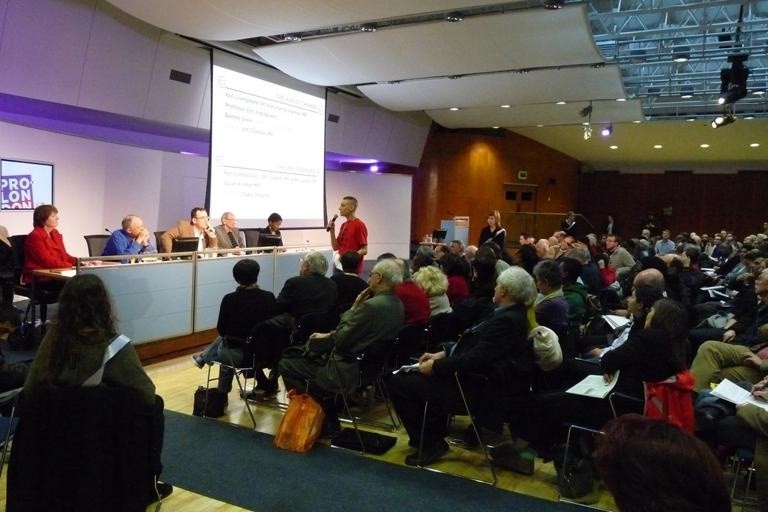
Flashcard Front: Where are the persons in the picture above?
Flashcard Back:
[3,196,768,512]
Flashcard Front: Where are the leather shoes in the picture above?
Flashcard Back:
[405,439,449,466]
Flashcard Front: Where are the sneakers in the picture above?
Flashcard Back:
[240,386,278,402]
[191,352,204,369]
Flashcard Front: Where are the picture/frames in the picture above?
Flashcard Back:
[0,158,54,211]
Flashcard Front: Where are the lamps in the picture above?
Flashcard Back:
[718,3,750,106]
[446,11,464,23]
[543,0,563,9]
[631,50,646,64]
[711,102,736,129]
[579,99,593,141]
[671,37,690,63]
[359,23,379,32]
[284,32,304,41]
[648,86,660,99]
[679,84,695,100]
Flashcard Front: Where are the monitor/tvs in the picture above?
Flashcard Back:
[433,230,447,243]
[258,234,281,253]
[172,237,199,259]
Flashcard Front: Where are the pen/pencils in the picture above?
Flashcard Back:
[751,383,768,396]
[410,357,419,360]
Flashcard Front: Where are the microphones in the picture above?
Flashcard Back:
[105,229,138,263]
[326,214,338,231]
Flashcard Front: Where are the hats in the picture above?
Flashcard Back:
[233,259,260,284]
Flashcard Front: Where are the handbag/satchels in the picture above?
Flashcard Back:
[193,385,225,419]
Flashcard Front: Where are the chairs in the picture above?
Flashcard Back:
[245,229,263,247]
[154,232,167,252]
[5,235,68,335]
[0,386,26,478]
[84,235,112,257]
[240,230,247,247]
[7,385,163,511]
